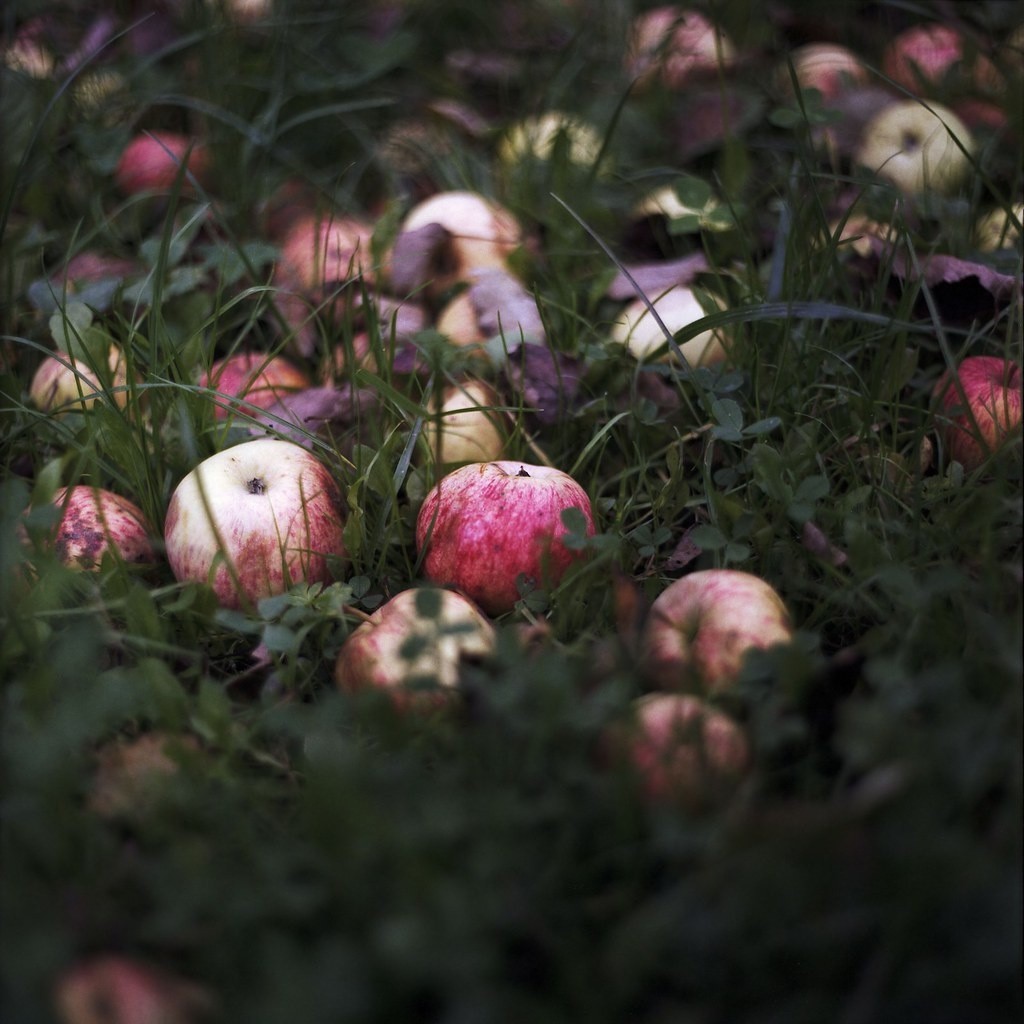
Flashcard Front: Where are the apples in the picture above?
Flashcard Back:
[29,6,1024,1024]
